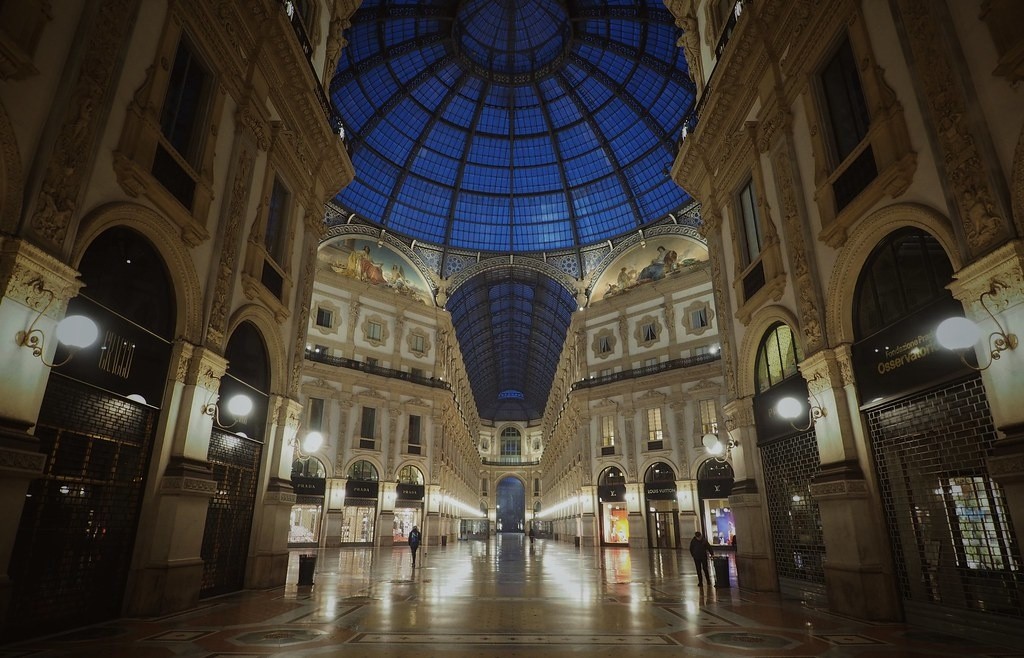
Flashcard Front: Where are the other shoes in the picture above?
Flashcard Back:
[412,563,415,567]
[707,580,711,585]
[698,583,703,587]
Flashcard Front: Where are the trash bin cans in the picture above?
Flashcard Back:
[442,536,447,546]
[575,537,580,547]
[710,556,731,588]
[296,554,318,586]
[555,533,558,540]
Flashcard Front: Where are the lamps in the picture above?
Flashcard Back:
[777,375,828,432]
[935,289,1018,370]
[287,414,323,461]
[15,278,99,368]
[702,419,739,463]
[201,370,253,428]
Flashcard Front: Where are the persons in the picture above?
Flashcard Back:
[409,526,422,567]
[690,532,715,587]
[529,528,535,543]
